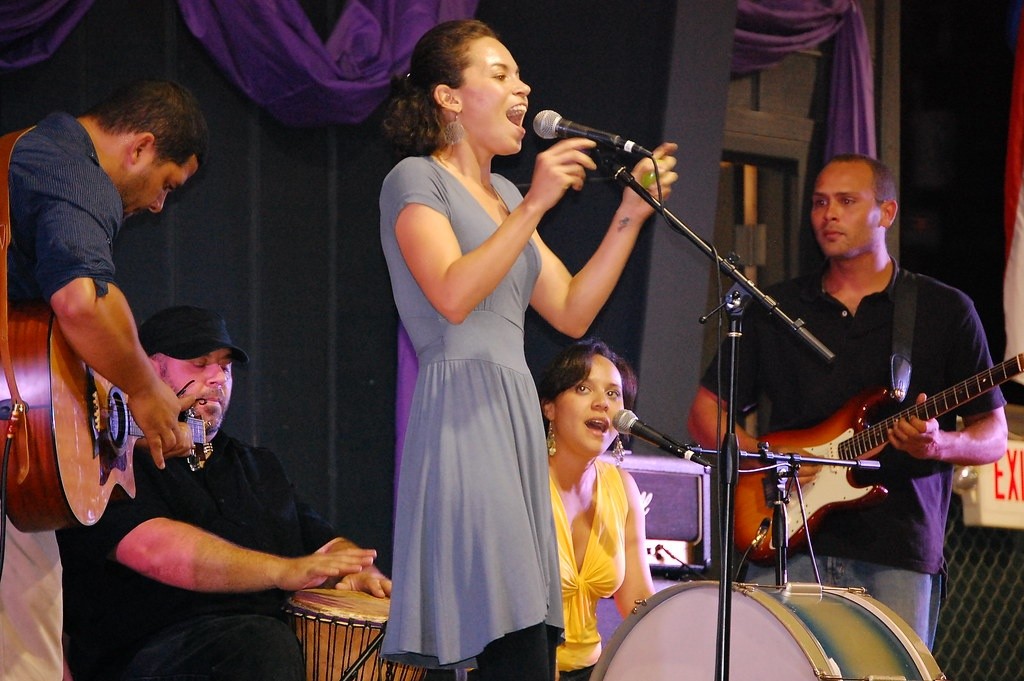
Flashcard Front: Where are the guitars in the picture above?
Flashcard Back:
[0,300,214,532]
[732,352,1023,567]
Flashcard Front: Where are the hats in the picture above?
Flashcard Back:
[138,305,251,365]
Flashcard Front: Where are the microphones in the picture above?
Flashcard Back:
[532,110,653,160]
[612,409,711,467]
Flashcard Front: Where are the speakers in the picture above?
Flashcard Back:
[594,451,713,572]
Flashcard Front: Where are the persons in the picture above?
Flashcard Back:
[363,18,680,680]
[52,303,393,680]
[535,338,658,680]
[0,76,211,681]
[688,150,1010,657]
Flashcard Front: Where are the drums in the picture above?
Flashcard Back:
[282,588,431,681]
[589,580,949,681]
